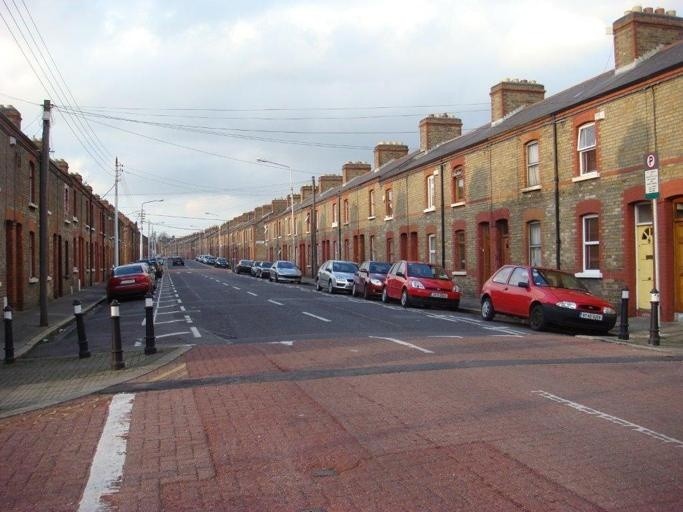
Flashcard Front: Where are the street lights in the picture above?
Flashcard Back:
[256,157,296,269]
[138,198,164,262]
[204,212,221,258]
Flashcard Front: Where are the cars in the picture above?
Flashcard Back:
[350,260,393,299]
[478,263,617,335]
[314,259,359,295]
[106,254,185,304]
[381,259,460,310]
[194,254,228,269]
[268,260,301,284]
[234,259,273,279]
